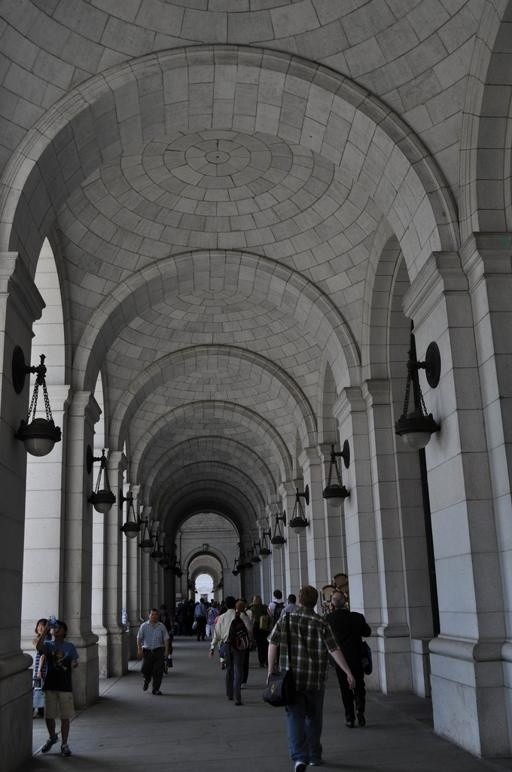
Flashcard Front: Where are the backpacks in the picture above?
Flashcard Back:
[272,602,285,626]
[227,611,251,651]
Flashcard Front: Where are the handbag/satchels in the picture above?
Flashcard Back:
[361,640,373,674]
[261,669,297,708]
[259,614,274,631]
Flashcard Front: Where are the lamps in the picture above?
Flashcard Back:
[394,341,441,449]
[288,484,310,534]
[322,439,350,507]
[120,486,182,584]
[12,346,62,457]
[86,444,116,514]
[233,527,272,575]
[272,510,287,550]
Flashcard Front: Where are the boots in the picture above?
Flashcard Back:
[219,658,225,670]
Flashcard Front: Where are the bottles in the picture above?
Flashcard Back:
[49,615,60,630]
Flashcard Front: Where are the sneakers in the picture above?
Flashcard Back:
[343,717,366,728]
[308,760,321,766]
[293,760,306,772]
[143,678,162,696]
[226,682,248,706]
[41,734,58,753]
[60,743,71,757]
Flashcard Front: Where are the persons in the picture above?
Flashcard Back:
[32,619,52,719]
[36,618,79,757]
